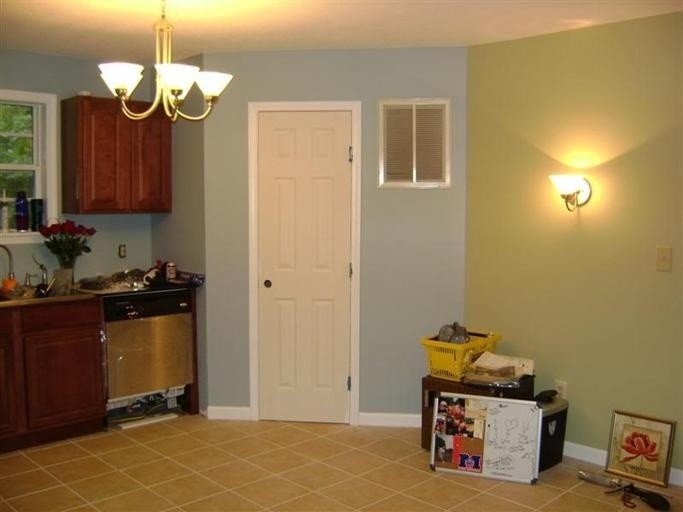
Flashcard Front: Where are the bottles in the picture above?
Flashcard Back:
[31,198,44,232]
[14,191,29,232]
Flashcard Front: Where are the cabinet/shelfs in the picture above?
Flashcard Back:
[61,95,172,214]
[0,299,107,454]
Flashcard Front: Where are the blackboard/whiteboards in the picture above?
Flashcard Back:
[429,392,543,484]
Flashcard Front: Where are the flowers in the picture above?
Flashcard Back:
[38,219,97,269]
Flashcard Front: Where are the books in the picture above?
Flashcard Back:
[464,348,535,384]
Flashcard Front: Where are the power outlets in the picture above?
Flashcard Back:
[554,380,567,400]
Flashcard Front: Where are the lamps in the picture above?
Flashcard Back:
[96,0,234,123]
[547,172,590,211]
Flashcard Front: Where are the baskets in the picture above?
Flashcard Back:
[419,329,502,382]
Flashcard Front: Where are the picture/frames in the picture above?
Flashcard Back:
[603,409,678,488]
